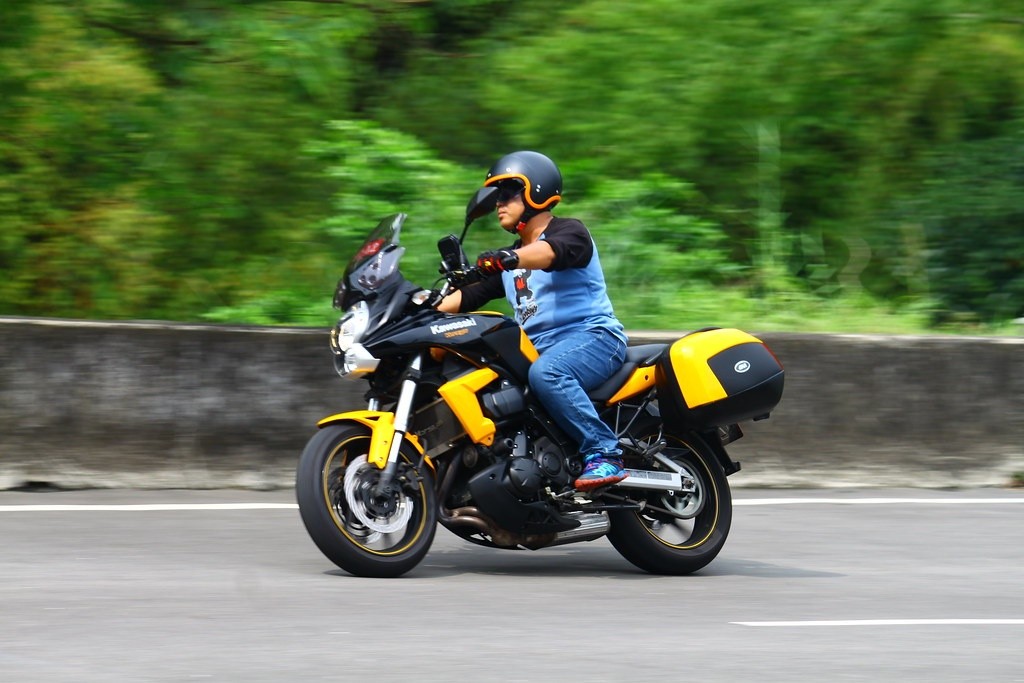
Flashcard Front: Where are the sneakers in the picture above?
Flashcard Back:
[575,451,630,492]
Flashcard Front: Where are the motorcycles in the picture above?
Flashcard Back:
[296,185,789,576]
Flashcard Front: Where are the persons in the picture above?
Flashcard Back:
[437,149,631,491]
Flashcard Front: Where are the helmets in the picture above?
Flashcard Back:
[483,151,562,211]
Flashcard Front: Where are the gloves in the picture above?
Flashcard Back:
[476,248,519,278]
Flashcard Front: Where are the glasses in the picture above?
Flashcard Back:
[494,181,526,202]
